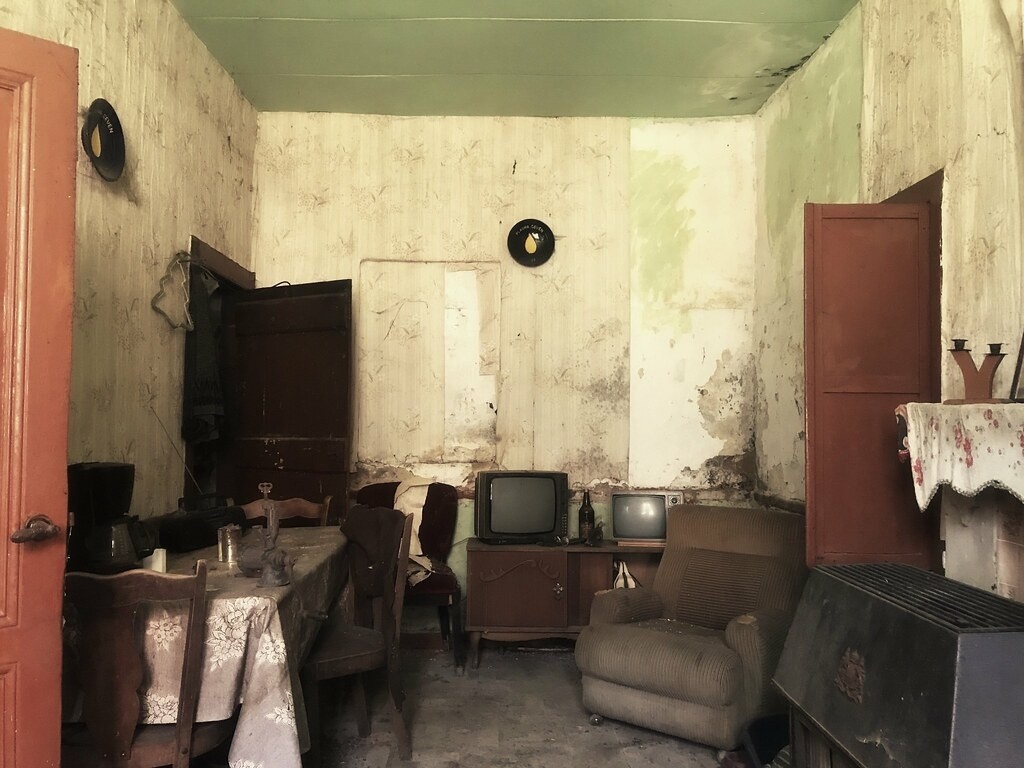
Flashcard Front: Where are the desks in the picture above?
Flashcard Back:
[61,523,349,768]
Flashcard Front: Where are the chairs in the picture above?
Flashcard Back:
[60,481,461,768]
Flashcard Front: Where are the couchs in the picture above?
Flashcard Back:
[577,504,805,764]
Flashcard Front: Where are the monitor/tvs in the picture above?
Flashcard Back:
[610,490,684,542]
[475,471,569,545]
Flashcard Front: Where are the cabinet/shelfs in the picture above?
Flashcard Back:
[465,538,664,684]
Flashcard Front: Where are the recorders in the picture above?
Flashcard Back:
[151,406,247,554]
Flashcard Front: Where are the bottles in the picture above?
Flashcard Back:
[579,488,595,542]
